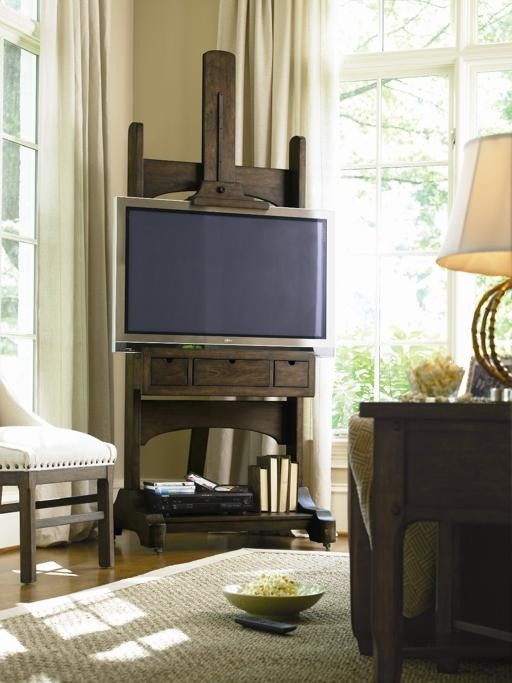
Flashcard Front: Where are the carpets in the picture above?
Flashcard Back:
[0,547,512,683]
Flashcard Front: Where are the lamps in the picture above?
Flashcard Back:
[435,133,512,402]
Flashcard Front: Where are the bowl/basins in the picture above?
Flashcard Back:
[405,364,466,402]
[220,579,328,621]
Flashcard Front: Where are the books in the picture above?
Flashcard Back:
[465,356,511,397]
[289,461,300,513]
[142,470,244,495]
[271,452,291,514]
[256,454,279,513]
[246,463,268,513]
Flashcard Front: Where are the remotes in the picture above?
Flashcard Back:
[235,616,298,635]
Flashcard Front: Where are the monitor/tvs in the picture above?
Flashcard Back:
[112,196,335,354]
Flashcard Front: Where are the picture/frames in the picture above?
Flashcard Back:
[468,354,511,400]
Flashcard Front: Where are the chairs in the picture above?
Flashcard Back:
[0,380,118,585]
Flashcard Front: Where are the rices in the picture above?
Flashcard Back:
[240,575,297,597]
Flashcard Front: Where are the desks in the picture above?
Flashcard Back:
[111,50,336,557]
[358,401,512,683]
